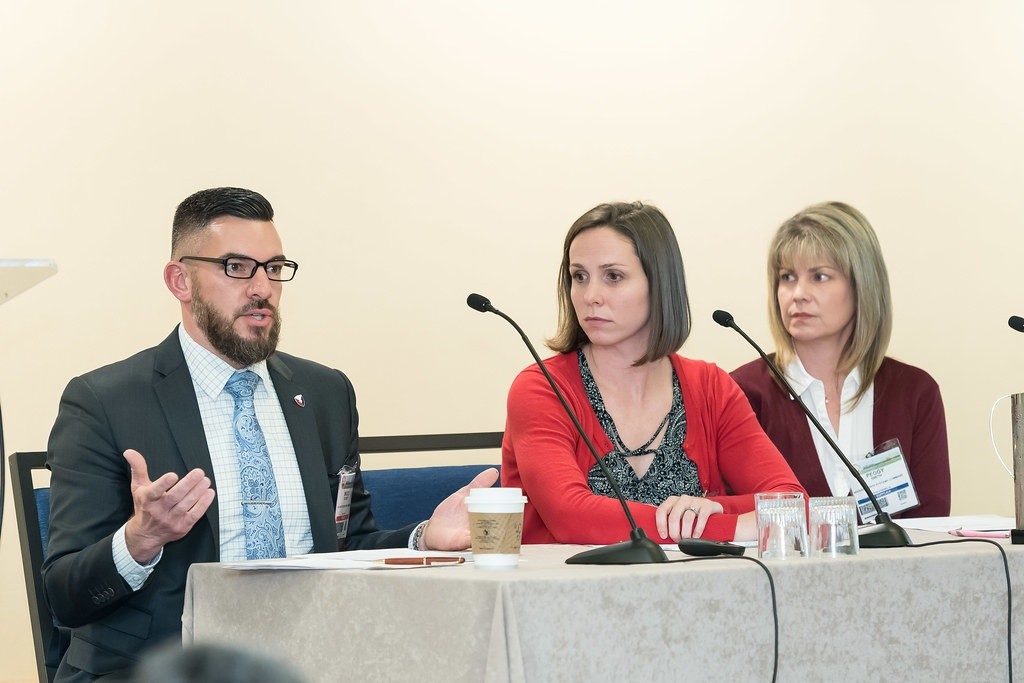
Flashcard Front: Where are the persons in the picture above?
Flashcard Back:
[44,187,500,681]
[499,203,811,543]
[725,203,950,525]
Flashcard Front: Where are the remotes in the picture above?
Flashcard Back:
[678,537,745,557]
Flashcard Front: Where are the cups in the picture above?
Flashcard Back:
[464,488,528,565]
[809,497,859,559]
[758,499,807,560]
[754,492,804,524]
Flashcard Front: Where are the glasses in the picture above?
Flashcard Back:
[180,253,296,281]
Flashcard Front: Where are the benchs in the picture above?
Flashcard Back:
[8,429,514,683]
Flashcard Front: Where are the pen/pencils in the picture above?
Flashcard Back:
[383,556,465,566]
[947,531,1010,538]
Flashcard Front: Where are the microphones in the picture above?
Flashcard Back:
[466,293,669,564]
[1008,316,1024,332]
[712,310,912,548]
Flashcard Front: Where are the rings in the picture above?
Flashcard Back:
[685,507,697,514]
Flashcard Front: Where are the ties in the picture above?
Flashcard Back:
[222,369,287,560]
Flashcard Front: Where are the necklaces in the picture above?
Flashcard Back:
[825,397,828,403]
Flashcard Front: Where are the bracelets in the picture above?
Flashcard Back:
[413,524,425,551]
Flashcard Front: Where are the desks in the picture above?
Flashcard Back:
[183,543,1024,683]
